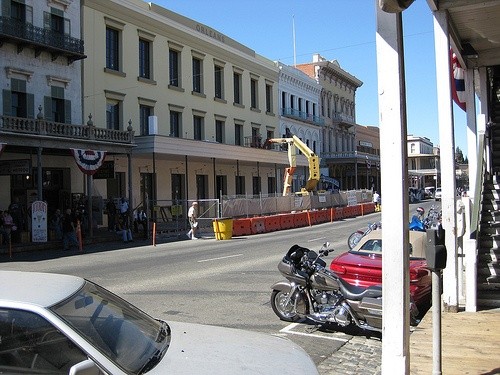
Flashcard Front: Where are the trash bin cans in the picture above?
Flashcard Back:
[211,215,234,241]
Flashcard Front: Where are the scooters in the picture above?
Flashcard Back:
[347,209,442,250]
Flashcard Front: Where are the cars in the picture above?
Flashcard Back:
[409,187,435,203]
[330,228,442,312]
[0,270,321,375]
[434,188,442,201]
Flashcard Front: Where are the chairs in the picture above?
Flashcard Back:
[0,316,100,375]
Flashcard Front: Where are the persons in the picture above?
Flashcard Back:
[116,197,128,215]
[106,197,116,232]
[114,215,133,243]
[187,202,199,239]
[134,207,147,239]
[0,196,26,245]
[374,191,379,207]
[50,208,82,251]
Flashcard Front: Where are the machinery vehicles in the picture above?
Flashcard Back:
[244,127,326,196]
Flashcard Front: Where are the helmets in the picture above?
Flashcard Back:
[416,207,424,214]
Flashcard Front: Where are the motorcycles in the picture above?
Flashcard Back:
[270,242,419,339]
